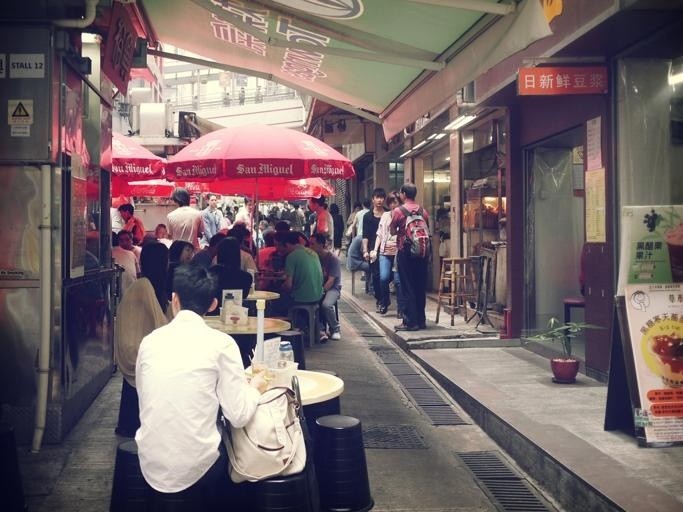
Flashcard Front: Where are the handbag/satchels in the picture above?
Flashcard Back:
[221,383,307,485]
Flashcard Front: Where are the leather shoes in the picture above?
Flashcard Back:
[419,321,425,330]
[394,323,418,331]
[376,302,390,314]
[397,311,404,319]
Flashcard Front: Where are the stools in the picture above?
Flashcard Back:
[314,413,375,512]
[0,424,28,512]
[113,375,140,437]
[351,268,369,296]
[435,257,484,326]
[563,296,585,355]
[288,304,320,345]
[245,474,311,512]
[267,330,305,370]
[108,439,160,511]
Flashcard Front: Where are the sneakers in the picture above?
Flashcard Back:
[319,331,328,342]
[331,332,340,340]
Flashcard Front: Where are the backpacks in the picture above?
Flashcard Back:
[400,206,432,260]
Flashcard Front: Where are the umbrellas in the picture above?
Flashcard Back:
[110,123,355,210]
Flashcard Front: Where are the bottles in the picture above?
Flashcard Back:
[281,341,294,362]
[223,293,234,321]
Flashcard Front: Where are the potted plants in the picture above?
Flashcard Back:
[522,317,608,383]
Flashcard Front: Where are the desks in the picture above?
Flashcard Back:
[245,369,345,418]
[203,316,291,336]
[242,289,280,317]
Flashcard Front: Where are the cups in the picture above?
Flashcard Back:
[250,362,268,394]
[666,242,683,285]
[647,339,683,389]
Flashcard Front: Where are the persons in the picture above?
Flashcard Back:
[345,182,435,330]
[238,88,246,106]
[108,180,344,342]
[257,86,263,103]
[226,93,232,107]
[134,265,270,498]
[193,96,199,111]
